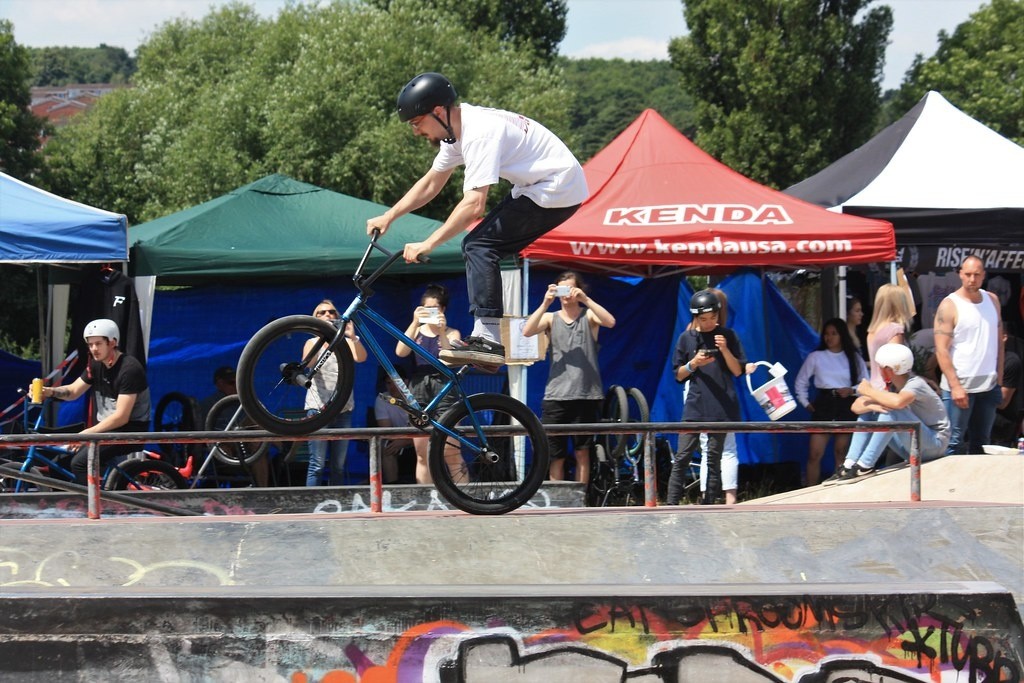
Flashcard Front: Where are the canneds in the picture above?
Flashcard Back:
[31,378,42,404]
[1018,438,1024,455]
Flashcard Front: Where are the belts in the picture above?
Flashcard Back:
[817,389,839,396]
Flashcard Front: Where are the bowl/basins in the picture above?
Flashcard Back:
[982,444,1019,455]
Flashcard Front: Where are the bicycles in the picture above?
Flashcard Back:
[236,228,550,515]
[0,388,272,493]
[590,385,722,506]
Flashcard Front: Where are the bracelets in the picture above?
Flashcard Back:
[352,336,359,343]
[686,362,694,372]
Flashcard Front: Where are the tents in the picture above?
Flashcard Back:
[783,90,1024,320]
[519,107,897,479]
[0,171,130,274]
[126,173,520,426]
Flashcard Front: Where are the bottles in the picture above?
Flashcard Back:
[32,378,42,404]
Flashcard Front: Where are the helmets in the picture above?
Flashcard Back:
[875,343,914,376]
[82,318,120,347]
[689,289,722,314]
[397,72,457,122]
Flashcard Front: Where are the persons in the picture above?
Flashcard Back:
[933,255,1004,454]
[667,287,756,506]
[795,317,869,486]
[302,300,367,487]
[822,344,952,486]
[866,283,913,389]
[198,367,304,487]
[375,364,416,484]
[847,298,866,361]
[28,319,151,485]
[396,285,469,484]
[522,272,616,481]
[990,335,1024,447]
[909,329,938,376]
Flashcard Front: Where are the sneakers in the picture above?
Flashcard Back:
[833,462,878,484]
[437,336,505,366]
[822,465,852,485]
[450,338,500,374]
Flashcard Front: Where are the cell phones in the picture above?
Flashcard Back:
[418,308,440,324]
[704,349,718,358]
[552,286,571,296]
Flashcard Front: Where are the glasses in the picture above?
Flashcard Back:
[409,112,428,128]
[317,309,337,315]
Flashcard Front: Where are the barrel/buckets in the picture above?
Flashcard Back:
[746,360,797,421]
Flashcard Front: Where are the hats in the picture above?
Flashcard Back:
[214,367,236,385]
